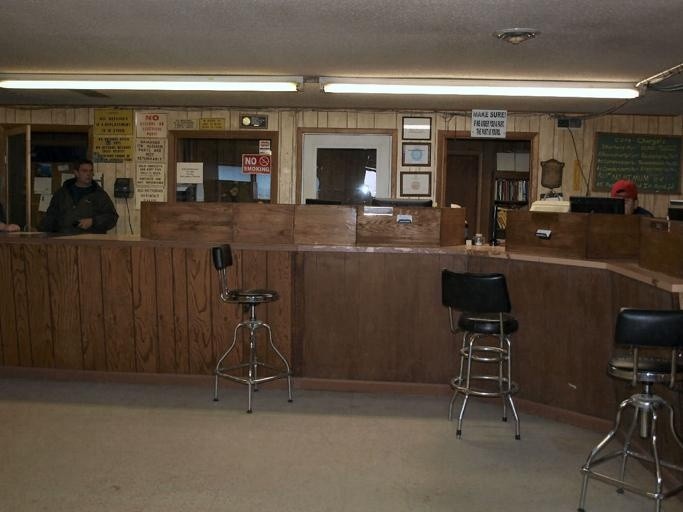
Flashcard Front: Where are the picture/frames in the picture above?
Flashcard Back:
[401,116,431,140]
[401,142,431,167]
[399,170,431,197]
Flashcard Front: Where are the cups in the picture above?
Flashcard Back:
[466,239,473,250]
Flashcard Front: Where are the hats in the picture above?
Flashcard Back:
[610,179,638,199]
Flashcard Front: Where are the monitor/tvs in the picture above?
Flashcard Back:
[372,198,433,207]
[570,196,625,215]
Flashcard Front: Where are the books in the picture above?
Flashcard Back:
[668,200,683,221]
[494,179,528,202]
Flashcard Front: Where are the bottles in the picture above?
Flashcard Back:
[474,233,483,246]
[489,231,497,246]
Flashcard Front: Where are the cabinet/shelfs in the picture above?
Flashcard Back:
[488,175,527,244]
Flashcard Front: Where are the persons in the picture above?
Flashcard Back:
[221,184,240,202]
[39,159,119,235]
[0,222,21,232]
[611,179,655,218]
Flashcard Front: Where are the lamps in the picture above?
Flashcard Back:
[0,73,306,93]
[319,77,645,99]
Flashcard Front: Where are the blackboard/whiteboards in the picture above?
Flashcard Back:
[592,131,683,194]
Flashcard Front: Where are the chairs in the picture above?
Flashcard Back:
[440,269,524,441]
[210,242,293,416]
[574,306,682,511]
